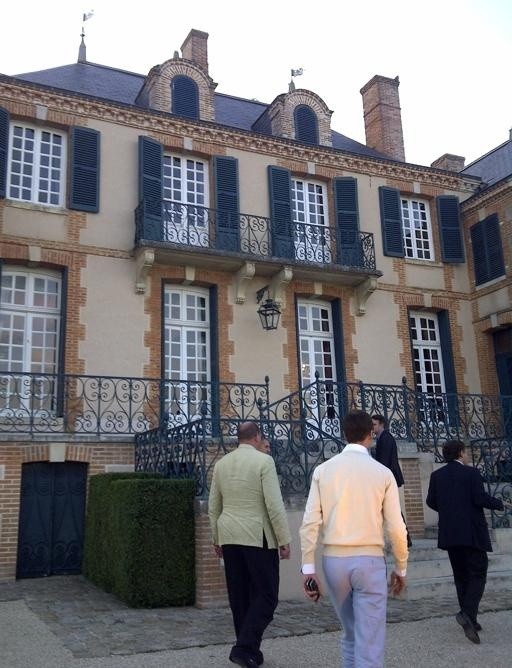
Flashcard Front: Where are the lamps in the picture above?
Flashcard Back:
[253,282,280,332]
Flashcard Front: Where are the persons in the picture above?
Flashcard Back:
[298,411,409,668]
[372,414,412,551]
[258,439,270,454]
[425,440,512,644]
[208,422,292,668]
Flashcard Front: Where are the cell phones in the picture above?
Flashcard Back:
[306,577,320,602]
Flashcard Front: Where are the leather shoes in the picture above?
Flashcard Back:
[456,611,482,643]
